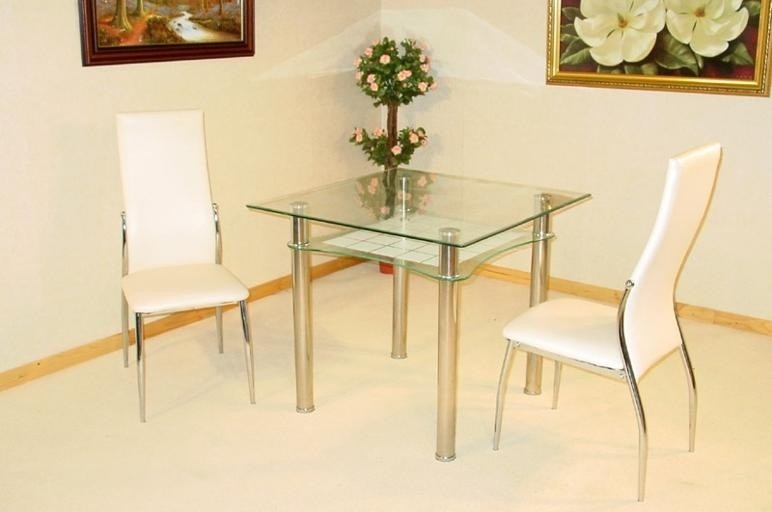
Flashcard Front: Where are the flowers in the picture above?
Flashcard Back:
[348,36,437,168]
[351,170,432,223]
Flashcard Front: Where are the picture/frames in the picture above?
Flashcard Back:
[546,0,772,99]
[79,1,255,66]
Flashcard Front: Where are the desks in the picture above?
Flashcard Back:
[245,169,594,466]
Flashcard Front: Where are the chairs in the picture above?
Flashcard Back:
[490,140,725,505]
[114,104,259,424]
